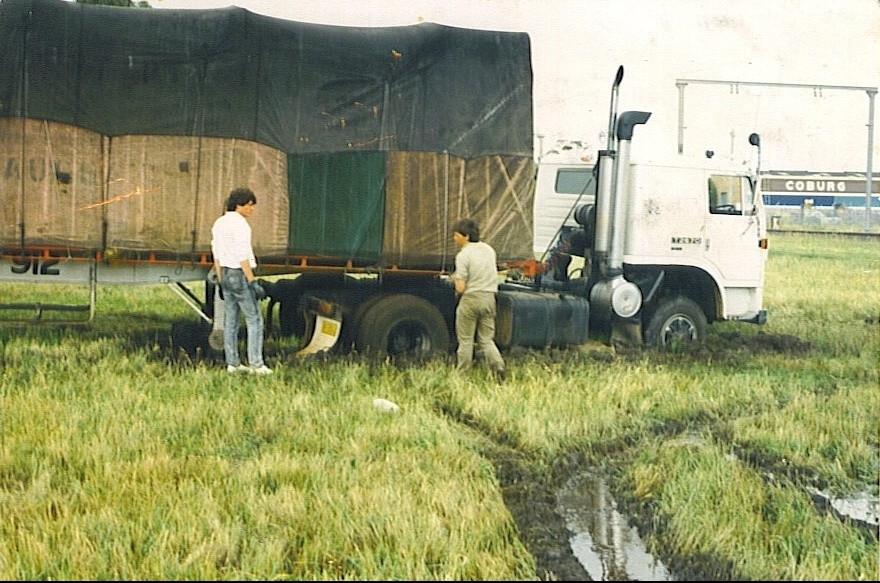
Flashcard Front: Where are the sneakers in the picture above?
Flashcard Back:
[226,363,247,374]
[246,365,272,375]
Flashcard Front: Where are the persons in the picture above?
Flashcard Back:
[452,220,508,385]
[211,187,275,374]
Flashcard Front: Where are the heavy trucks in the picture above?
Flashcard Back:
[3,1,775,363]
